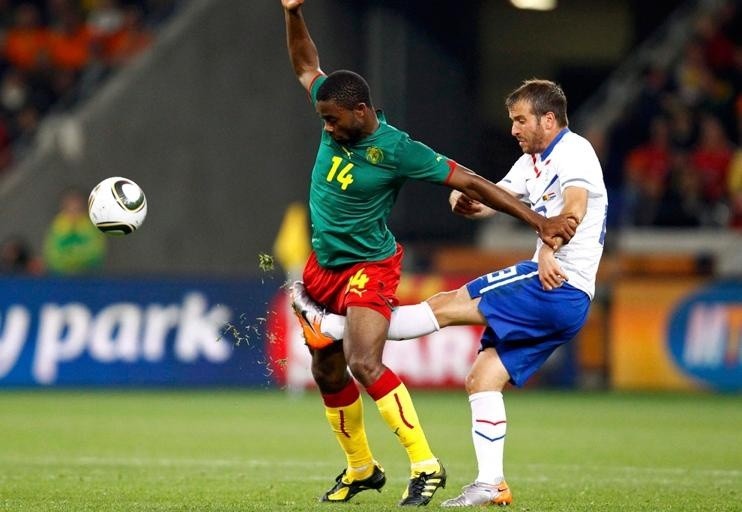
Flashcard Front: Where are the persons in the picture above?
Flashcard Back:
[0,0,168,176]
[276,0,579,507]
[288,77,613,506]
[618,8,738,231]
[0,233,40,274]
[39,187,108,276]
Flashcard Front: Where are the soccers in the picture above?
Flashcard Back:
[88,177,148,236]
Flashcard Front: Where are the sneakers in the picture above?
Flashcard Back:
[398,457,448,508]
[288,280,334,353]
[321,458,387,503]
[440,479,513,509]
